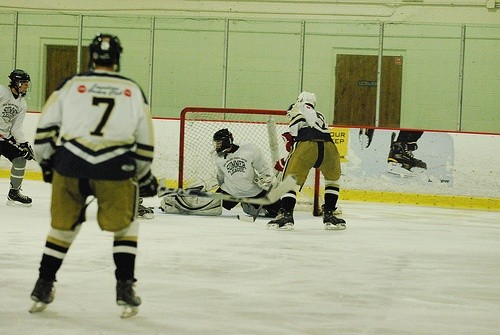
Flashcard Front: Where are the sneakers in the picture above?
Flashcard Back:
[116,277,141,318]
[30,277,56,312]
[386,132,427,176]
[7,186,32,207]
[358,128,374,152]
[268,207,294,229]
[321,205,346,229]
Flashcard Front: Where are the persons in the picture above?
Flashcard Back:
[190,91,346,227]
[0,70,34,204]
[29,33,154,306]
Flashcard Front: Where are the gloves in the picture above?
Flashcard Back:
[282,133,293,152]
[19,142,34,160]
[40,159,53,183]
[274,158,284,172]
[138,170,157,196]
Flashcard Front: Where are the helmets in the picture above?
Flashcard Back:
[213,129,233,153]
[297,91,316,109]
[10,69,30,84]
[89,34,120,65]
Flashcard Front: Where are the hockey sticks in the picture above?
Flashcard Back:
[156,173,299,205]
[0,132,37,162]
[236,170,281,223]
